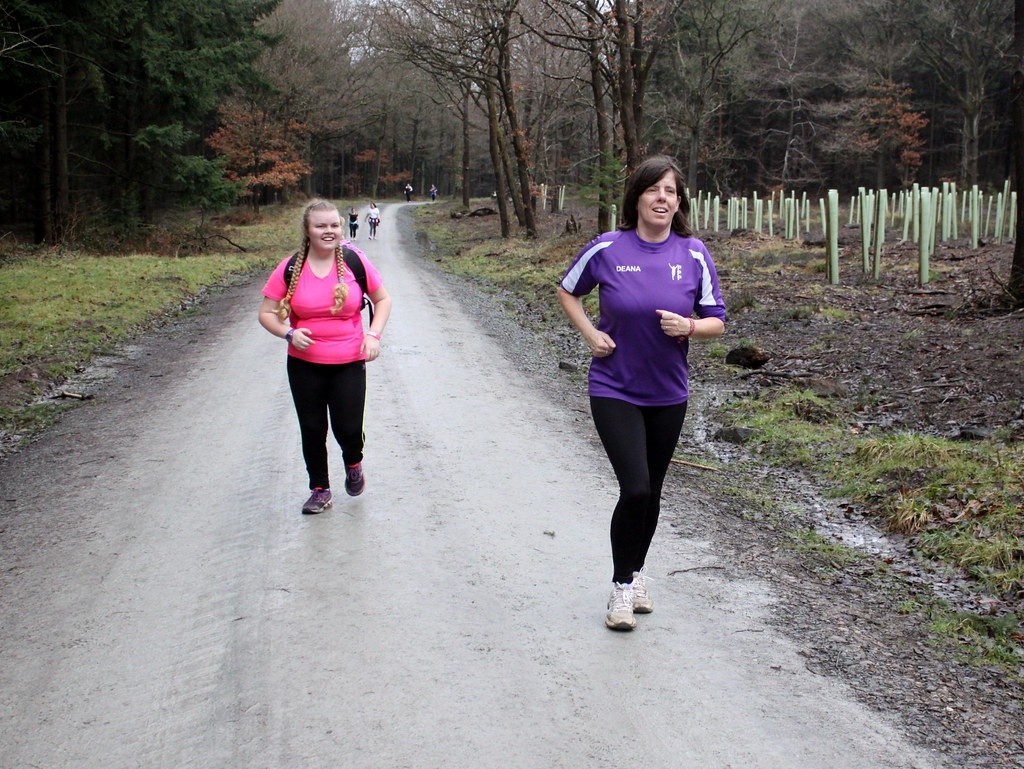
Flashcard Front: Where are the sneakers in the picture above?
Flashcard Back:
[301,487,333,515]
[344,459,364,498]
[606,571,652,631]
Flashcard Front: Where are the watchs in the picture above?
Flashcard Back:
[285,328,294,345]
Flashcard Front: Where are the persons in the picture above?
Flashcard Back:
[557,154,728,631]
[403,183,414,203]
[428,183,438,201]
[364,202,380,240]
[347,208,359,238]
[259,198,393,514]
